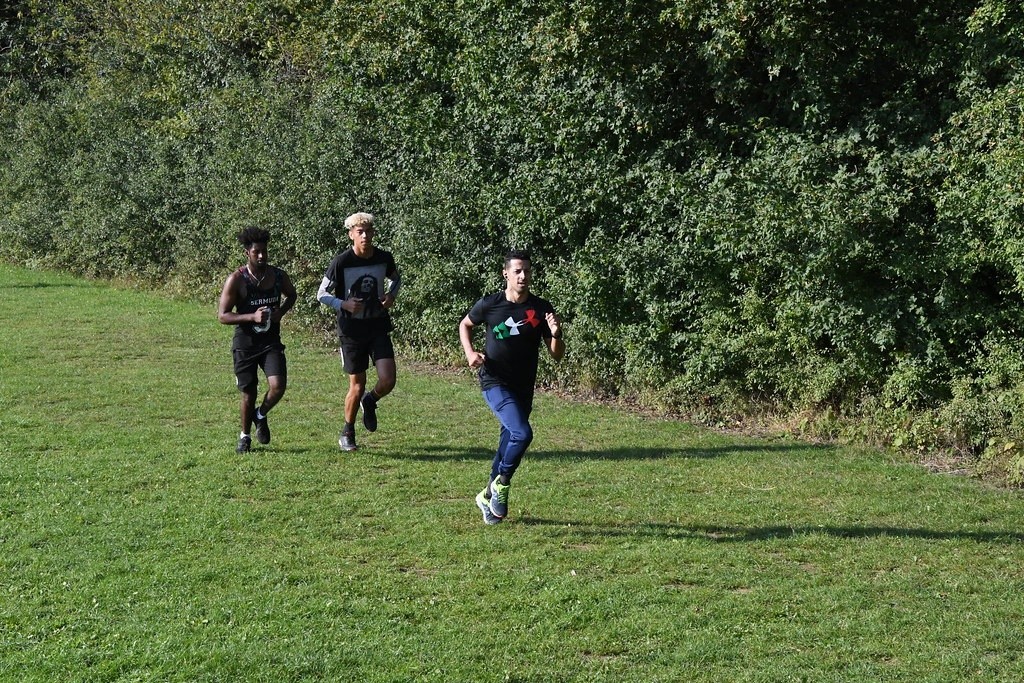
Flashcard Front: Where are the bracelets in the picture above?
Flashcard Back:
[550,329,563,340]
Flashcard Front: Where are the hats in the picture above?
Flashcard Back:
[344,211,374,229]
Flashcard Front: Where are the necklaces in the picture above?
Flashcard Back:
[248,263,268,287]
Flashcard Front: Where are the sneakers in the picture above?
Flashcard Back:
[236,436,252,454]
[475,486,502,525]
[253,407,270,444]
[490,474,511,518]
[360,391,378,432]
[338,425,357,451]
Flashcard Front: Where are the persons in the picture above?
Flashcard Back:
[317,212,400,451]
[460,250,566,526]
[218,226,298,454]
